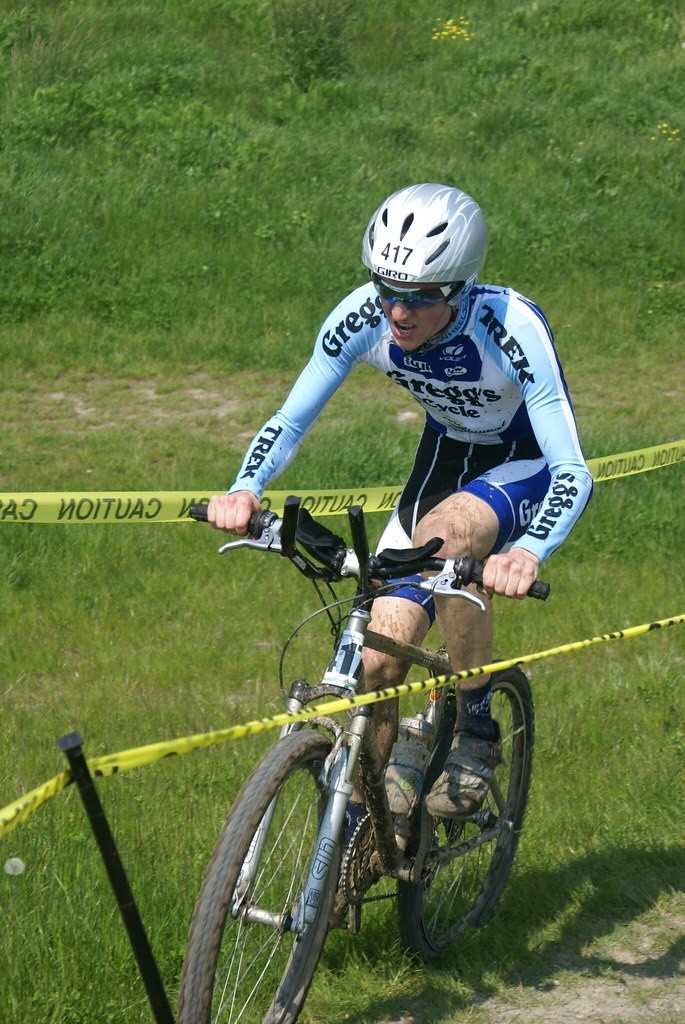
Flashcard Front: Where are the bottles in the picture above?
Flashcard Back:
[385,712,436,814]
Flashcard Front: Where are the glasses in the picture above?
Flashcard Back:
[370,275,458,307]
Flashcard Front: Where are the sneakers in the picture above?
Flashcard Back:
[424,712,504,817]
[313,845,368,928]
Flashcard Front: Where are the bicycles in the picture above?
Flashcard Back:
[176,492,552,1023]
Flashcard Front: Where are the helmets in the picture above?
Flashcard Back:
[360,183,489,307]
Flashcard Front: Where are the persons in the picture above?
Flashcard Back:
[209,181,596,930]
